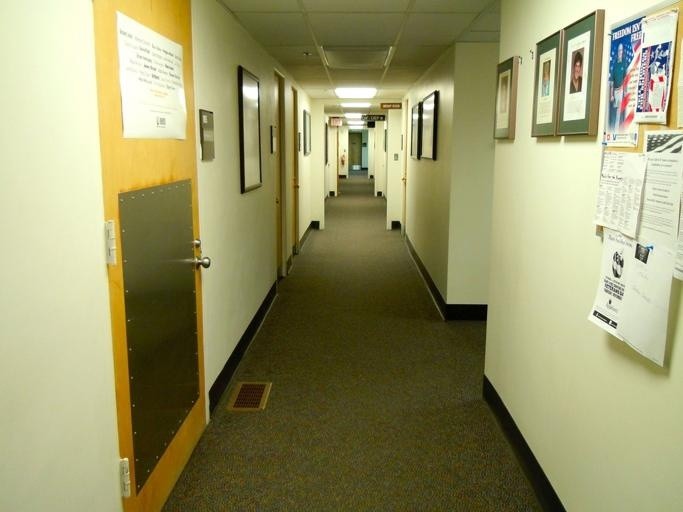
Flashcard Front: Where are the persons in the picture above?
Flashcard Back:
[569,51,583,95]
[541,63,550,97]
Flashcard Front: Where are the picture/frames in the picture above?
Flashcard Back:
[494,56,519,140]
[556,9,605,137]
[421,91,437,160]
[531,30,562,137]
[238,66,262,193]
[411,103,421,160]
[304,110,311,154]
